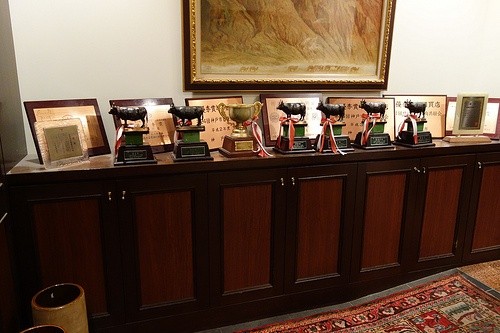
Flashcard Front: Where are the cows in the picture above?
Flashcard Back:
[404,99,427,120]
[316,100,346,121]
[276,99,306,121]
[359,98,388,118]
[169,103,204,126]
[108,102,149,128]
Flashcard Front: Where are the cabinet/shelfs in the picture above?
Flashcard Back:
[6,144,500,333]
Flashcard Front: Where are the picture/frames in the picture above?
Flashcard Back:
[181,0,396,93]
[23,93,500,171]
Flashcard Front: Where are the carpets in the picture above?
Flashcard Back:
[194,267,500,333]
